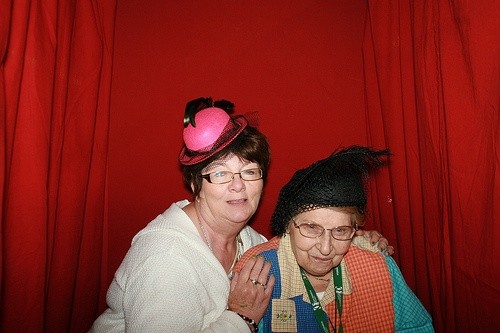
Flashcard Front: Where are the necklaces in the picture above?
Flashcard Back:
[194,202,213,253]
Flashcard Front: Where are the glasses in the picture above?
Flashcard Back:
[291,214,358,241]
[198,167,263,184]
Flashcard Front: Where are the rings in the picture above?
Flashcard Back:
[249,278,265,287]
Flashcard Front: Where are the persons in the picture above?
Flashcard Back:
[233,144,436,333]
[88,97,396,333]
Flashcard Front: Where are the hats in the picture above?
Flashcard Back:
[178,96,249,166]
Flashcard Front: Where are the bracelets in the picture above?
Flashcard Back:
[227,309,259,333]
